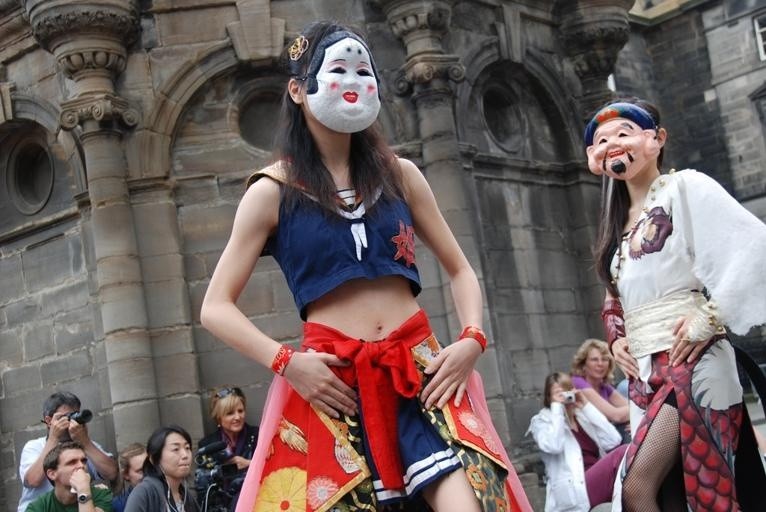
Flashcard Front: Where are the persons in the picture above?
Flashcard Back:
[20,440,114,511]
[15,391,120,511]
[522,372,640,512]
[197,18,537,511]
[107,441,151,511]
[193,382,261,512]
[579,93,765,512]
[122,424,204,511]
[566,338,640,446]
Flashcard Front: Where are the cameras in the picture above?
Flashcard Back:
[563,390,576,404]
[64,409,93,425]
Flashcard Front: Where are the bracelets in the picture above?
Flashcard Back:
[459,326,488,353]
[271,342,296,376]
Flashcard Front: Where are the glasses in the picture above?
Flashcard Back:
[215,387,243,398]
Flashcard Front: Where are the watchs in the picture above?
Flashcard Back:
[76,492,92,503]
